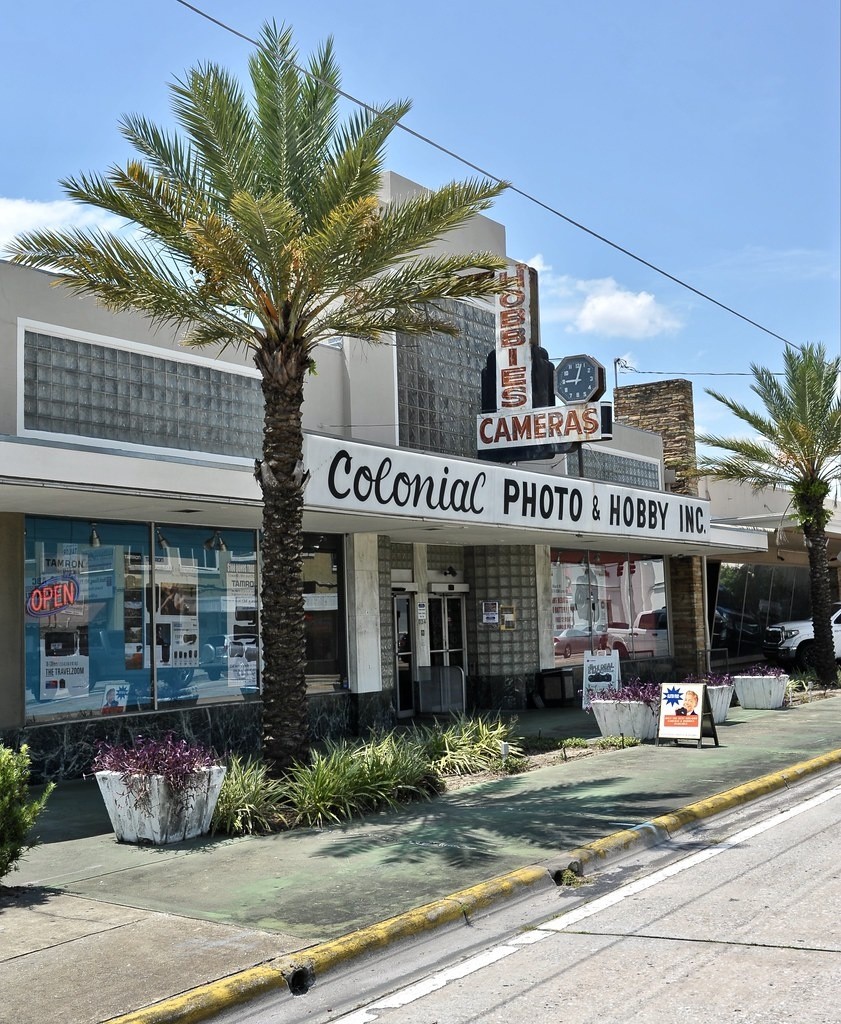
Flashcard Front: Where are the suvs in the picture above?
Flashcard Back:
[200,632,263,681]
[764,601,841,673]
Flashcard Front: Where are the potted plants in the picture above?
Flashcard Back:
[730,663,790,710]
[586,677,662,742]
[82,731,227,846]
[681,672,736,725]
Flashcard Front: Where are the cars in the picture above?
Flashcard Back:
[553,608,668,659]
[25,623,195,703]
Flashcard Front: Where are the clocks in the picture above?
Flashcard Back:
[554,354,606,406]
